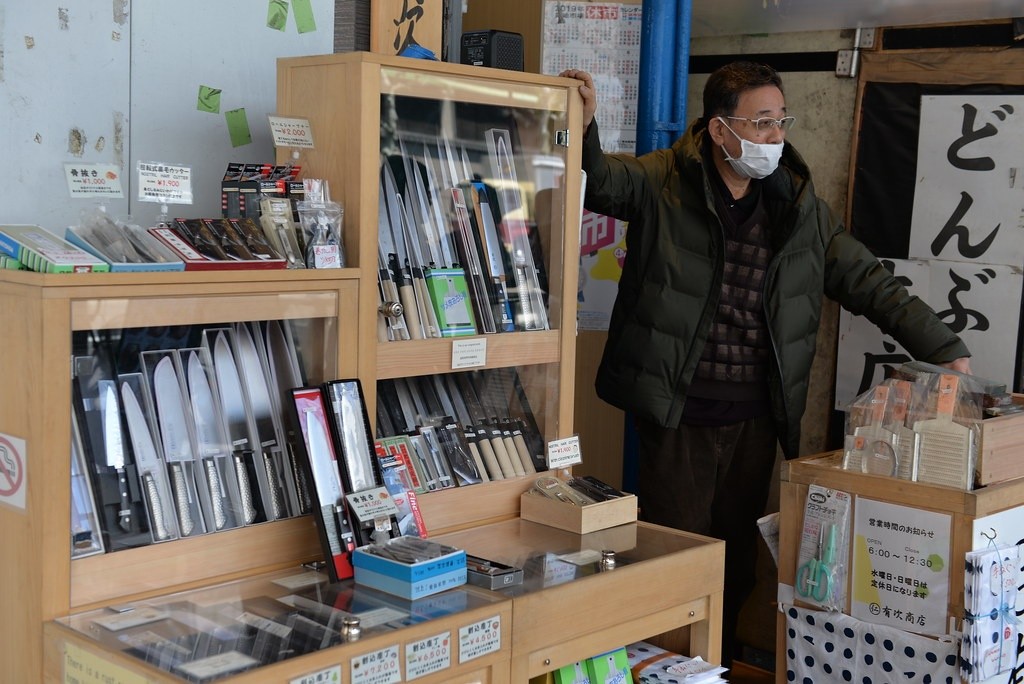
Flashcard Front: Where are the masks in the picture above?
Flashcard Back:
[718,117,784,179]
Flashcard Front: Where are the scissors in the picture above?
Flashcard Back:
[797,522,831,604]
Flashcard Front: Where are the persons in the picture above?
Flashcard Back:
[558,64,973,673]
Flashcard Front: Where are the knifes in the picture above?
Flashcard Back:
[100,130,540,542]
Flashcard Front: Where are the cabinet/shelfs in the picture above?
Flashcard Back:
[274,52,726,684]
[0,50,514,684]
[776,451,1024,684]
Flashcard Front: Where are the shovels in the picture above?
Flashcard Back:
[842,385,900,480]
[882,380,921,483]
[912,375,978,492]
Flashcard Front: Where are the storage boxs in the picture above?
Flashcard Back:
[847,391,1024,486]
[71,127,639,602]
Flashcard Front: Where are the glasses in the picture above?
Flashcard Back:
[725,116,795,134]
